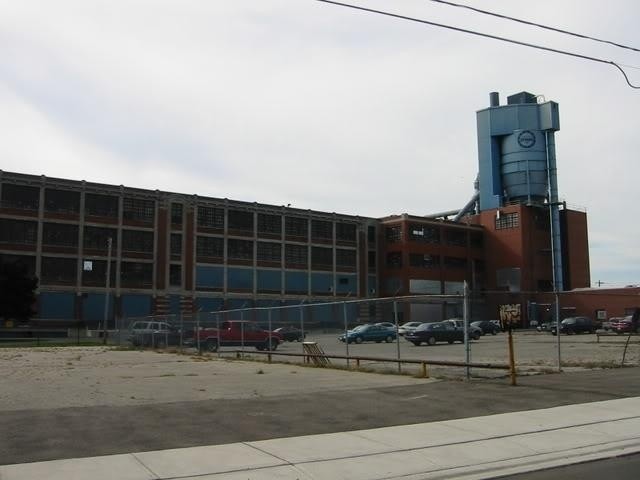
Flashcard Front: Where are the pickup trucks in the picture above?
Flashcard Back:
[194,320,280,352]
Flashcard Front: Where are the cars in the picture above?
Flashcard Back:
[338,317,500,346]
[273,326,306,342]
[537,310,640,336]
[125,320,194,347]
